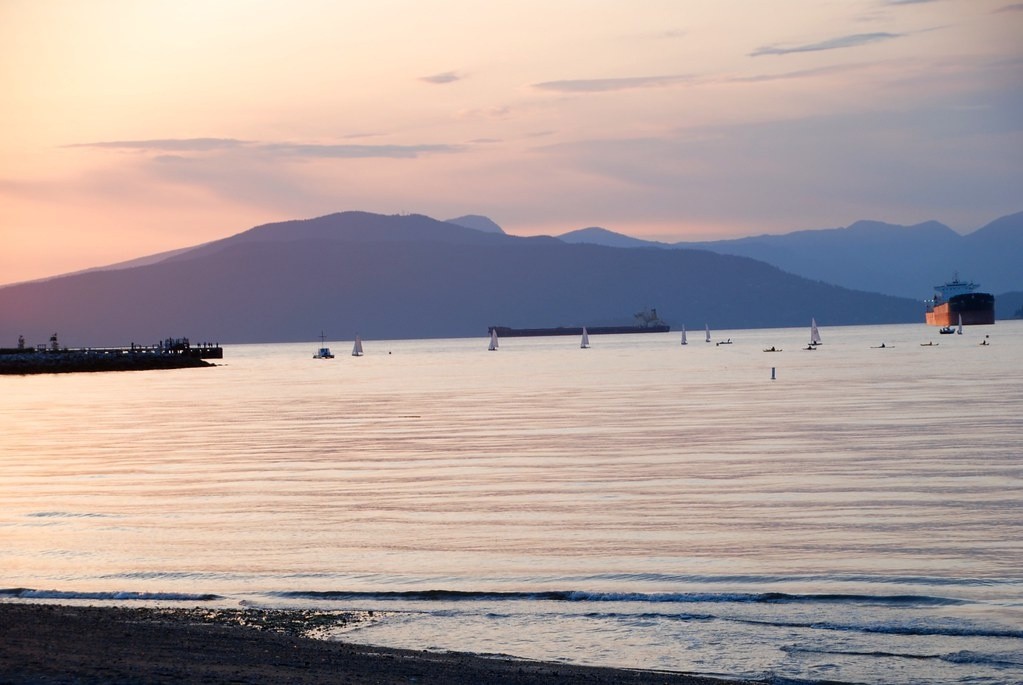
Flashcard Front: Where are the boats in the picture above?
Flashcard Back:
[920,272,996,327]
[802,347,817,351]
[938,327,956,334]
[980,343,990,345]
[312,346,335,359]
[920,343,940,347]
[763,349,783,352]
[717,340,733,345]
[871,346,896,349]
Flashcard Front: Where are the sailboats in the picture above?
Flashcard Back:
[957,314,964,335]
[351,335,365,357]
[806,317,822,346]
[705,323,712,342]
[680,324,688,344]
[580,326,590,349]
[487,328,500,351]
